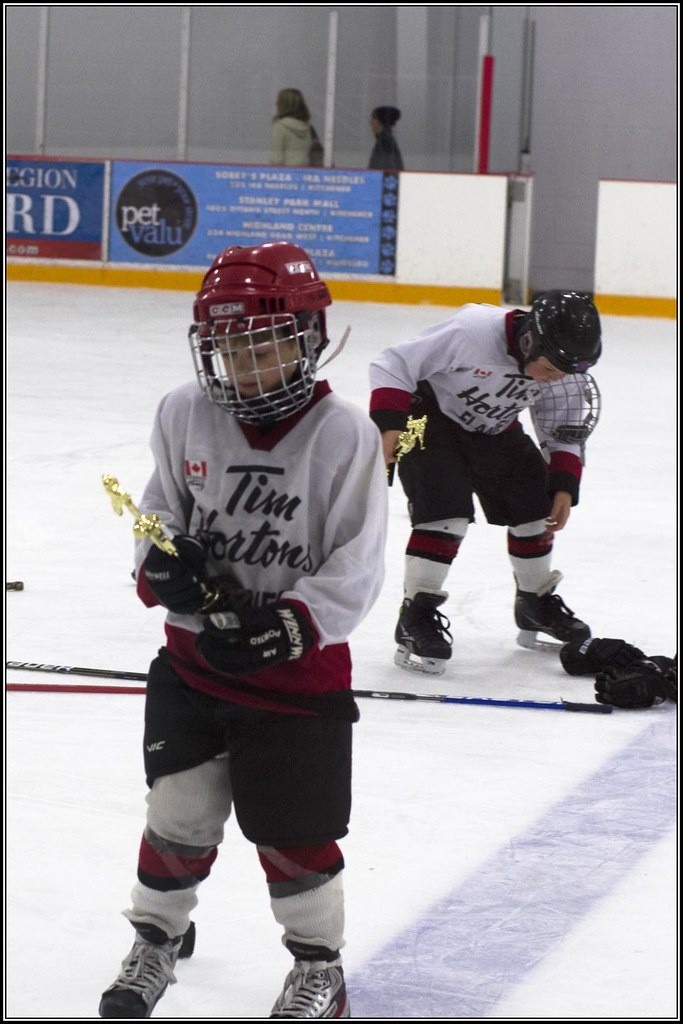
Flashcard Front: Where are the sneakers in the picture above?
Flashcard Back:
[269,933,350,1017]
[393,588,453,676]
[513,570,590,653]
[98,908,195,1018]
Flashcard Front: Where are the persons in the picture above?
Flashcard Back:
[366,106,405,170]
[368,290,601,676]
[270,88,312,166]
[98,241,392,1018]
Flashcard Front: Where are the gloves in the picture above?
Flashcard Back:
[559,638,647,676]
[595,655,678,709]
[196,601,315,676]
[143,535,210,614]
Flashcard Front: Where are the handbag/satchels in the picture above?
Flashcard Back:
[310,126,324,166]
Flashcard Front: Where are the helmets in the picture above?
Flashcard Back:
[516,290,603,374]
[188,242,331,424]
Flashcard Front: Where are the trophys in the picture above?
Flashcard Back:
[100,475,230,615]
[386,414,428,486]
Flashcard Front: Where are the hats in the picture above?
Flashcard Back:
[376,107,400,127]
